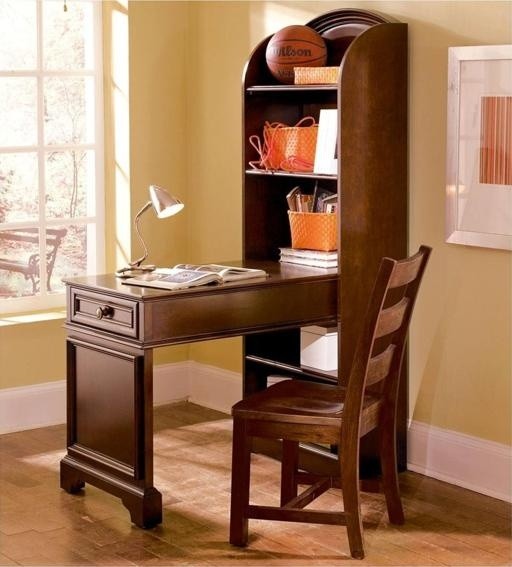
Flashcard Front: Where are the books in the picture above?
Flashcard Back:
[121,262,269,292]
[279,254,339,270]
[278,246,338,260]
[285,181,338,213]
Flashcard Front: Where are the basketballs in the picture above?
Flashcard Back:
[264,24,327,83]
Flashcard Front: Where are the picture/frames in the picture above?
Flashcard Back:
[441,42,512,251]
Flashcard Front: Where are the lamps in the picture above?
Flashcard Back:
[116,182,185,279]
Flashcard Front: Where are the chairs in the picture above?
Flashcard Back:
[227,243,434,558]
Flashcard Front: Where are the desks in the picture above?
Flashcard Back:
[59,262,340,533]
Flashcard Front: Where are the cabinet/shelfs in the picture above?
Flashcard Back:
[239,7,409,479]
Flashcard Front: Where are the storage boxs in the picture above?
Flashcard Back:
[298,322,338,371]
